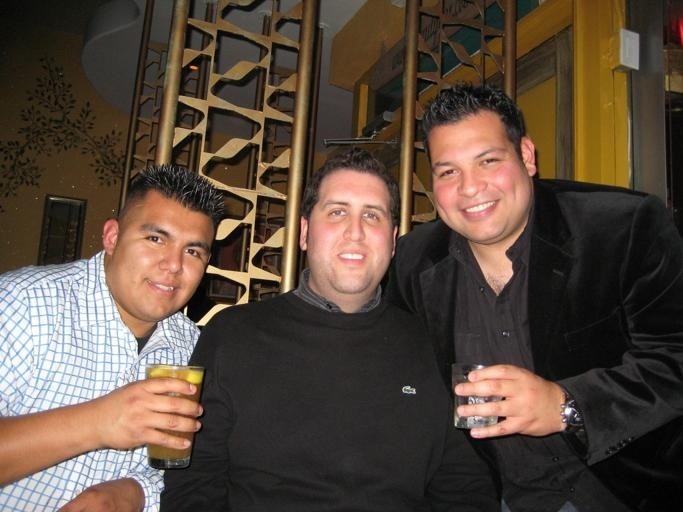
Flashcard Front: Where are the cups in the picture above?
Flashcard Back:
[452,362,502,430]
[143,364,205,471]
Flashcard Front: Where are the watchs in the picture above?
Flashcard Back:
[559,385,583,435]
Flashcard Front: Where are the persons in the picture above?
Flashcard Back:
[378,79,680,512]
[0,163,227,512]
[160,148,502,512]
